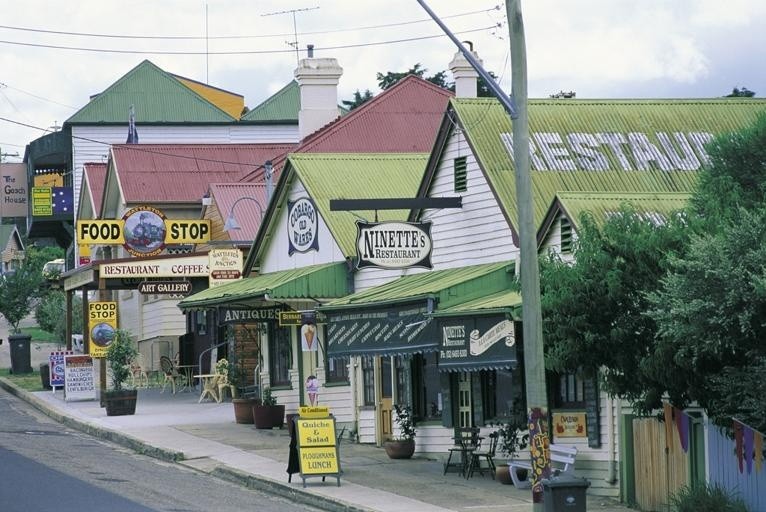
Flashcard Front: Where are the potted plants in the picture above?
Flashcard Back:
[383,403,417,460]
[489,394,528,485]
[101,326,138,416]
[216,357,286,431]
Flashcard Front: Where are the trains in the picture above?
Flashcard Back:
[132,214,164,242]
[94,329,112,344]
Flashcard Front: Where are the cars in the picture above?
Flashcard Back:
[2,269,26,281]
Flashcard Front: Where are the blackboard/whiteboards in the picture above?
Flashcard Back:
[64,354,95,401]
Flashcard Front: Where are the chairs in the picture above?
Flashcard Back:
[159,356,182,394]
[443,427,499,480]
[213,356,235,403]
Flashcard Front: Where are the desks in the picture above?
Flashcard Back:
[175,364,199,393]
[193,374,219,404]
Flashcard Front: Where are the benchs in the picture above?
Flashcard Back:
[505,443,579,489]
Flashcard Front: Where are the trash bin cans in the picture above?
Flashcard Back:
[8,334,33,374]
[542,477,590,512]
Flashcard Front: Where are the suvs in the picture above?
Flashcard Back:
[38,258,64,293]
